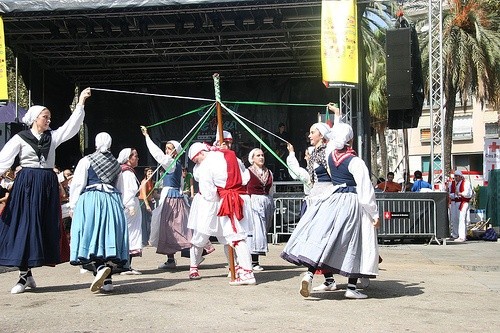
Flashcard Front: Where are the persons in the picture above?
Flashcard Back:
[0,87,91,294]
[450,170,473,243]
[140,125,215,269]
[280,103,382,298]
[114,148,143,275]
[180,166,194,207]
[374,170,432,192]
[240,148,275,270]
[0,167,74,246]
[138,167,157,248]
[69,132,131,294]
[187,130,258,285]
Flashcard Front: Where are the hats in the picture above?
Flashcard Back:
[187,142,208,160]
[23,105,46,127]
[116,147,132,164]
[168,140,183,154]
[454,169,462,176]
[330,122,353,150]
[216,130,233,143]
[95,131,112,154]
[248,147,259,165]
[313,122,331,140]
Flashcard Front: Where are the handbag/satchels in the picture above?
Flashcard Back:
[478,228,497,241]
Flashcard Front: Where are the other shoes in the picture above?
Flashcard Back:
[189,268,200,280]
[90,265,111,291]
[10,273,31,293]
[158,261,176,269]
[102,278,114,291]
[345,288,368,299]
[299,276,312,297]
[227,268,238,279]
[230,277,256,285]
[250,264,264,271]
[446,237,465,242]
[28,275,36,289]
[312,281,337,291]
[119,269,142,275]
[360,276,370,288]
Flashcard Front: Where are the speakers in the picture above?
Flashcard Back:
[0,122,27,150]
[385,28,424,129]
